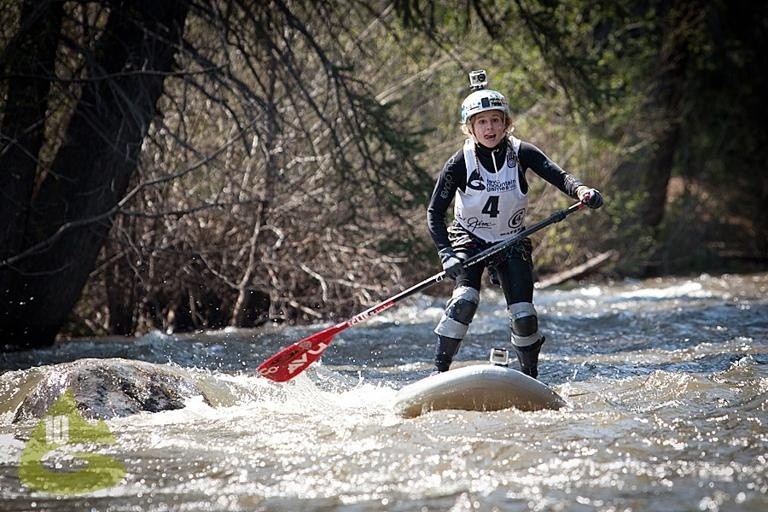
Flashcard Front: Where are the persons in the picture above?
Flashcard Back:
[423,87,606,383]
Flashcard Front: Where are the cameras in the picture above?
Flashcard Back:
[469,70,487,86]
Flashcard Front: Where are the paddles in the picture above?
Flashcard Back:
[257,193,592,383]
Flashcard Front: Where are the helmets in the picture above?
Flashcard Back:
[459,90,513,150]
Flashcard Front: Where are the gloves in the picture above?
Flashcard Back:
[576,186,605,211]
[438,247,463,281]
